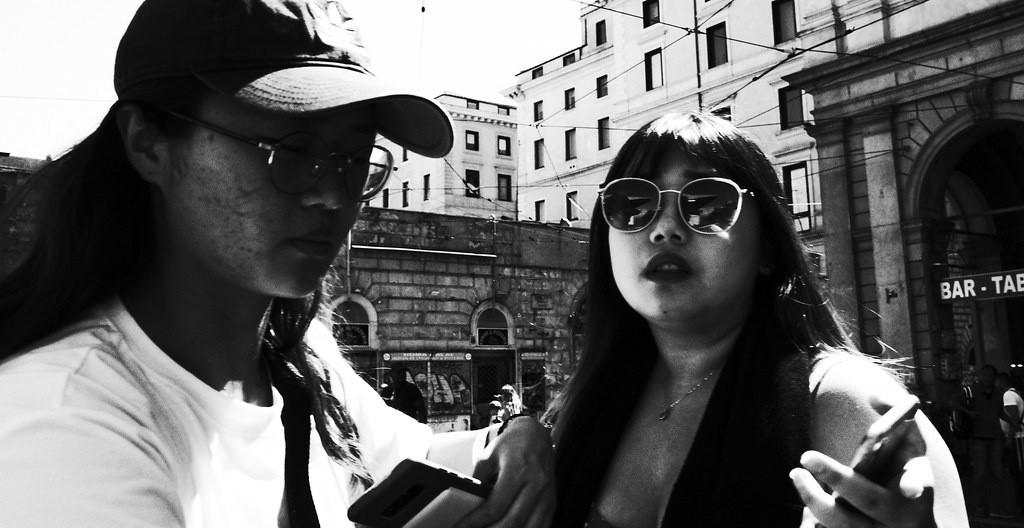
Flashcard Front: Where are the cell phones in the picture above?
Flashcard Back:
[347,458,492,528]
[830,393,921,513]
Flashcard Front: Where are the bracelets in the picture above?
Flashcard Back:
[497,413,535,436]
[472,427,488,470]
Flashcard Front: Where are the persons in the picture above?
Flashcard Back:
[538,110,973,527]
[348,356,557,424]
[0,0,557,528]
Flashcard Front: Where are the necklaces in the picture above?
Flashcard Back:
[657,359,720,420]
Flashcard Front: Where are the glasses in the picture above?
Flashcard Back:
[151,103,398,202]
[597,176,755,235]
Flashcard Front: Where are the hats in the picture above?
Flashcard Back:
[114,0,455,158]
